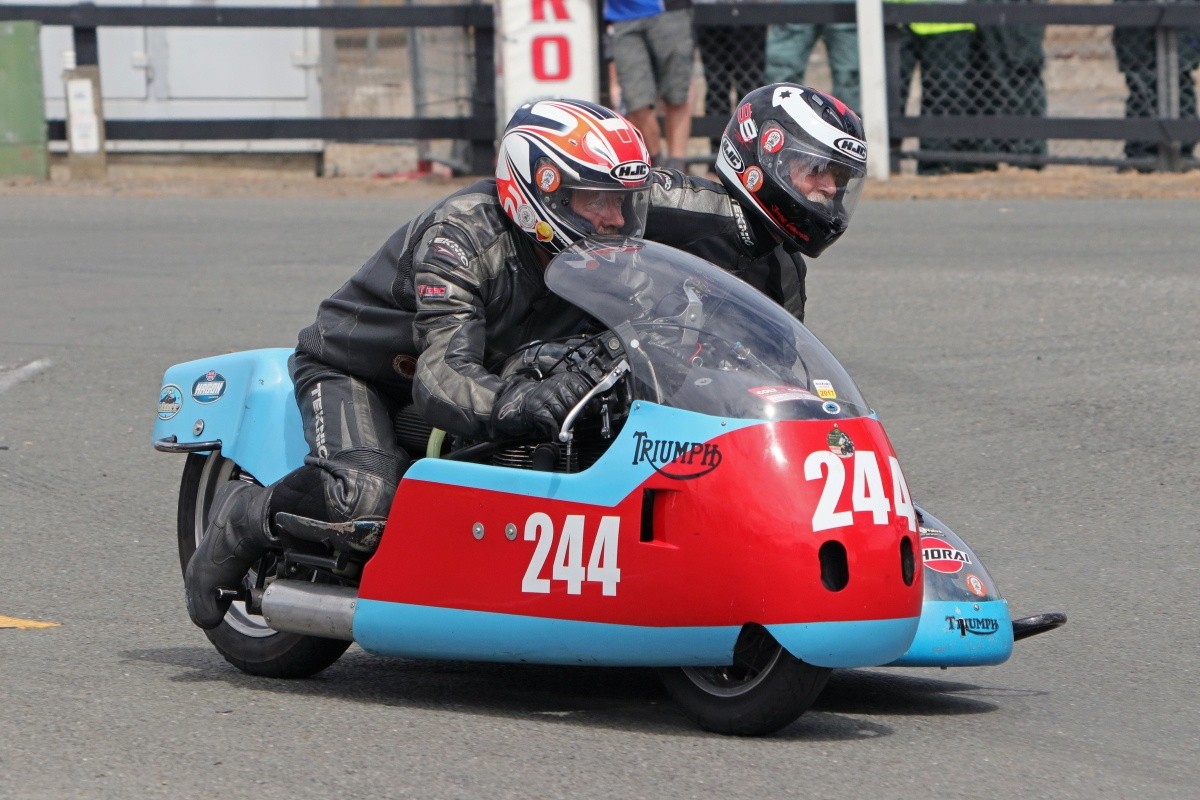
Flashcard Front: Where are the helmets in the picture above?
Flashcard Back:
[714,83,870,258]
[497,96,651,279]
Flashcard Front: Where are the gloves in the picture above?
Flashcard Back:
[485,374,600,443]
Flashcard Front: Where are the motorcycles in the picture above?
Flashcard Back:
[151,233,1070,746]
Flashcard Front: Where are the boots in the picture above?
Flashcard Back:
[185,481,278,628]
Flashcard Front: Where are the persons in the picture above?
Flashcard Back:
[187,81,871,627]
[599,0,1046,185]
[1111,0,1200,174]
